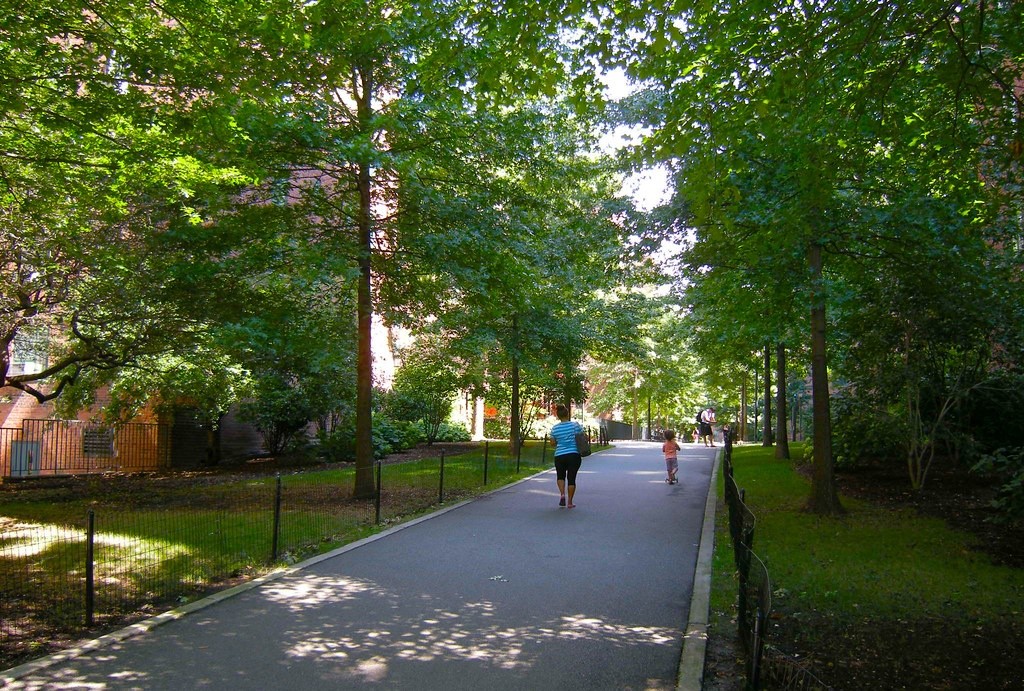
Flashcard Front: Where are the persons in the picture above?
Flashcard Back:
[699,407,717,447]
[723,422,731,448]
[549,405,584,508]
[662,430,681,484]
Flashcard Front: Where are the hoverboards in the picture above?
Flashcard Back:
[664,448,680,484]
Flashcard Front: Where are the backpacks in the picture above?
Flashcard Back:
[697,410,709,423]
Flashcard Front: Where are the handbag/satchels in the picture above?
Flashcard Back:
[574,422,591,457]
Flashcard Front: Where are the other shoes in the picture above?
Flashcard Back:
[670,472,675,481]
[559,495,566,506]
[567,504,576,508]
